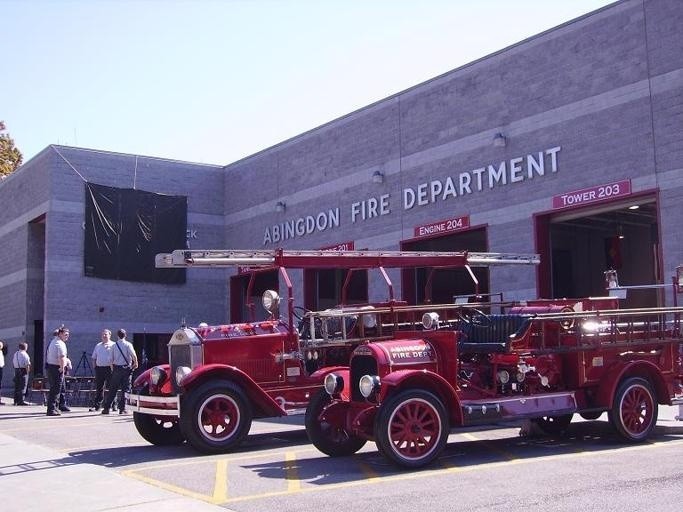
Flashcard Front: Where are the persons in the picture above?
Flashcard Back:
[0,341,6,406]
[101,328,138,415]
[88,328,118,413]
[12,343,32,406]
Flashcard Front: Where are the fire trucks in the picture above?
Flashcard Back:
[129,249,618,451]
[302,307,683,453]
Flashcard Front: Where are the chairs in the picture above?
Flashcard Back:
[27,374,121,412]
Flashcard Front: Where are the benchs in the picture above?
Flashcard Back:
[459,314,533,355]
[304,316,356,339]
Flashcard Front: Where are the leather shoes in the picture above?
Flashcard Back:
[18,403,127,415]
